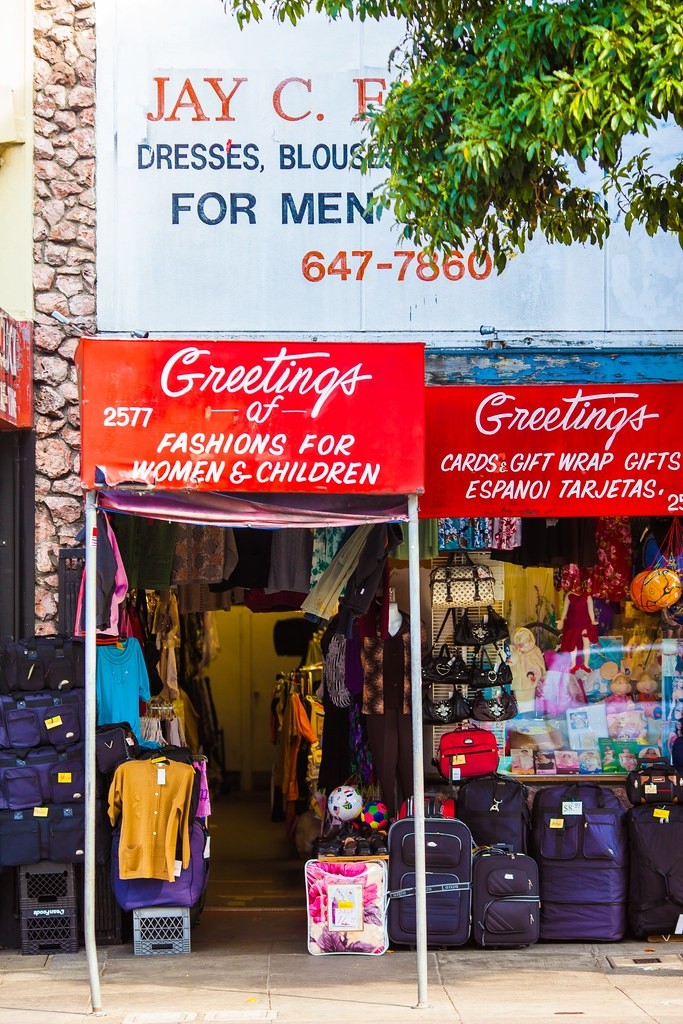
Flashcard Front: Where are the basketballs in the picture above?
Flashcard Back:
[629,569,661,613]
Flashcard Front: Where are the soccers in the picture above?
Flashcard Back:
[359,800,390,831]
[327,785,363,822]
[642,567,682,609]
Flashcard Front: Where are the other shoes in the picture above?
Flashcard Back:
[313,837,387,859]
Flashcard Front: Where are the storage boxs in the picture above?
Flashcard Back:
[19,860,78,953]
[133,907,190,955]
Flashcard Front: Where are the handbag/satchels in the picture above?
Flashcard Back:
[625,760,683,804]
[1,638,133,868]
[471,685,519,722]
[421,687,471,724]
[469,642,514,688]
[419,609,467,684]
[430,721,499,785]
[430,552,496,608]
[454,607,511,646]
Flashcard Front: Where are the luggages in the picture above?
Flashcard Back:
[387,792,473,949]
[532,780,628,944]
[471,842,540,949]
[627,757,683,940]
[455,773,531,855]
